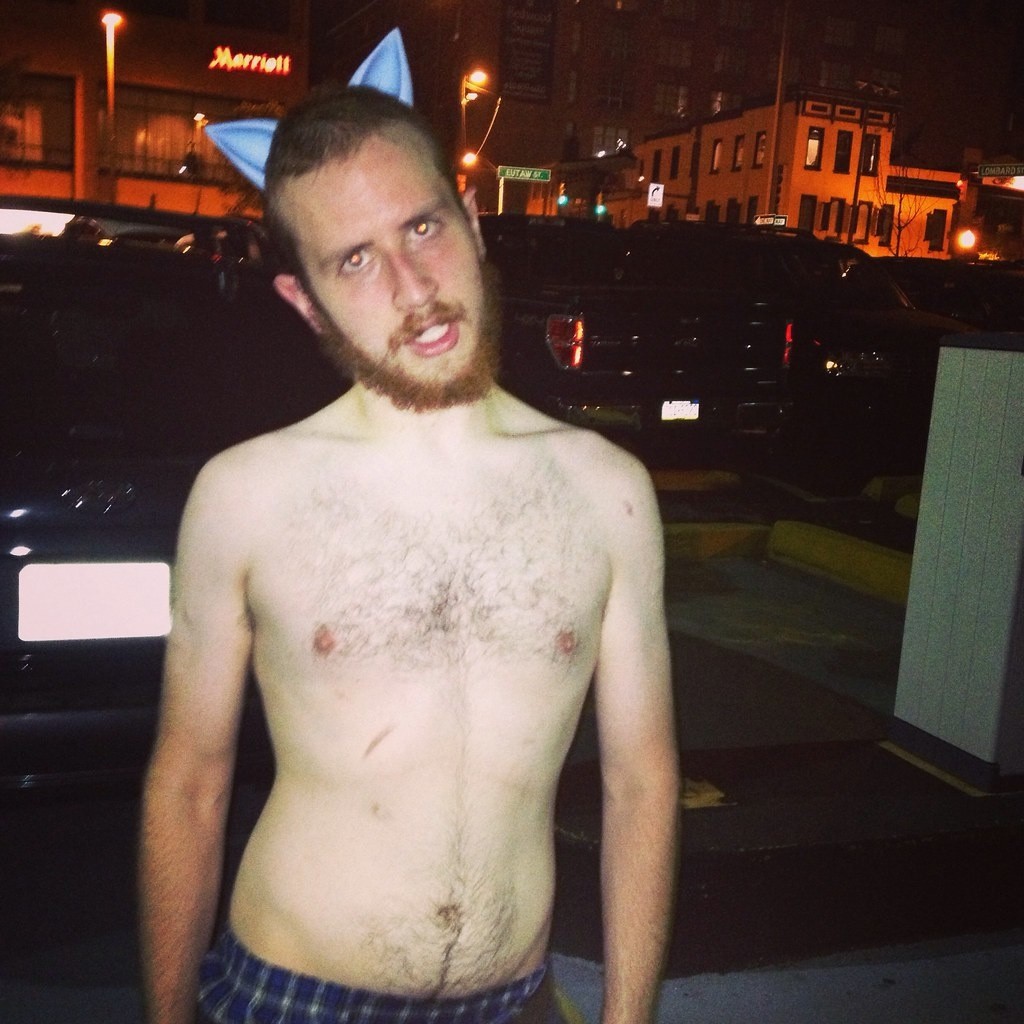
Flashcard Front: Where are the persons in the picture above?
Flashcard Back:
[134,29,676,1024]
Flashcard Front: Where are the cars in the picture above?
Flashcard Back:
[0,193,1024,541]
